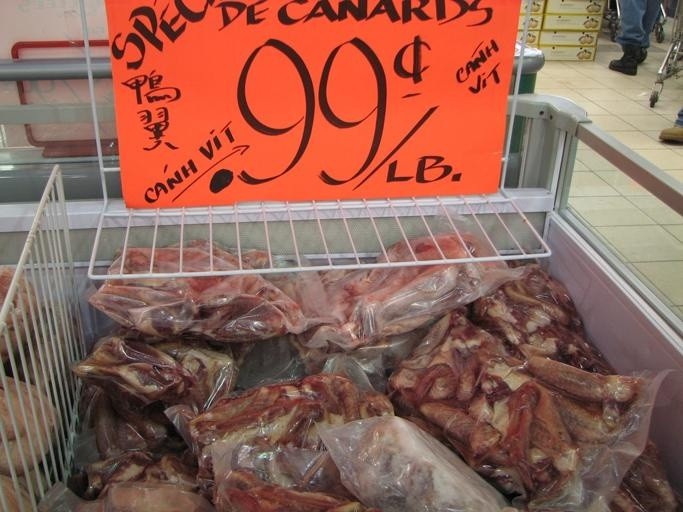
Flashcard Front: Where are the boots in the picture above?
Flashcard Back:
[608,43,647,75]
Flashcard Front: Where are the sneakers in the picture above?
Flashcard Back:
[659,124,682,143]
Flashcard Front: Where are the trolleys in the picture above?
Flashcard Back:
[604,0,667,43]
[649,0,683,108]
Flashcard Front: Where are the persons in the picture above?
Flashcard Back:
[659,106,683,144]
[608,0,665,76]
